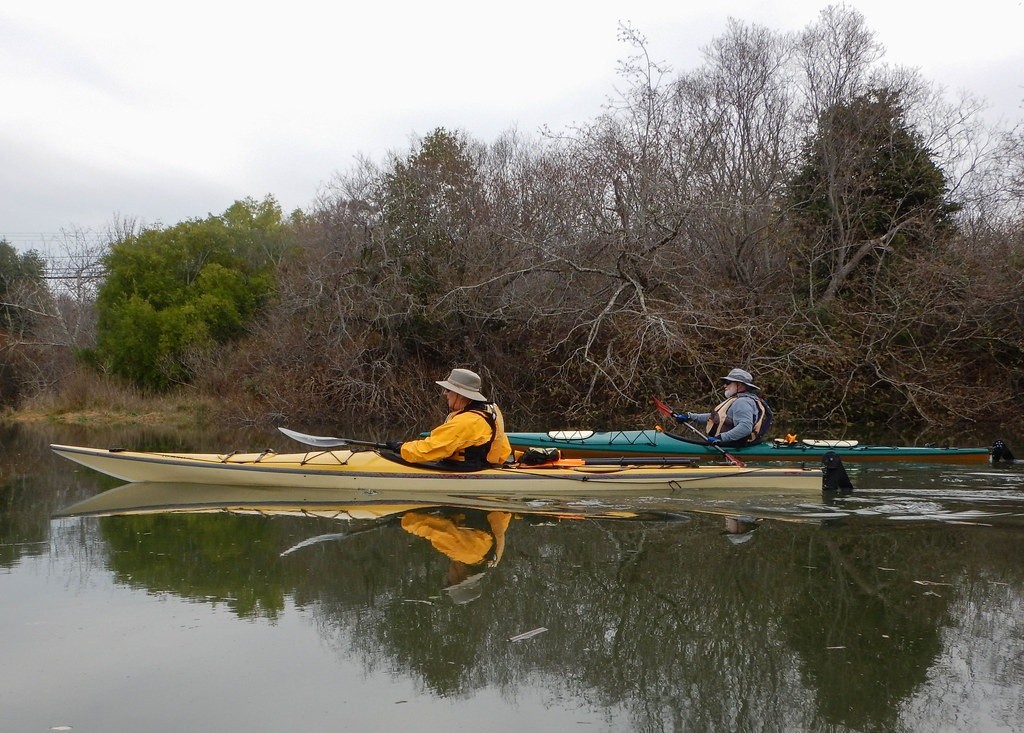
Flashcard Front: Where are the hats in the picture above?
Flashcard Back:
[720,368,760,389]
[436,367,486,401]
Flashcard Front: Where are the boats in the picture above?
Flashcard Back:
[49,483,833,522]
[419,426,1014,466]
[49,442,852,494]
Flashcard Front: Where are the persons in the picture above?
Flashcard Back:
[676,368,772,445]
[719,515,759,545]
[375,506,514,605]
[385,369,512,472]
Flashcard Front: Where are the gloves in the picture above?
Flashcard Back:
[386,440,403,453]
[670,411,690,425]
[708,437,723,445]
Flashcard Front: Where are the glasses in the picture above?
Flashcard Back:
[723,380,731,384]
[442,387,450,392]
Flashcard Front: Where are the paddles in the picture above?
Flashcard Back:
[278,427,405,447]
[650,393,747,468]
[279,519,397,557]
